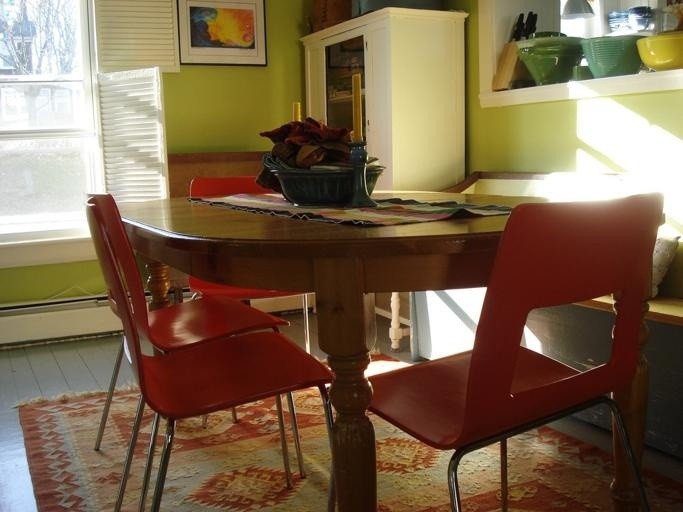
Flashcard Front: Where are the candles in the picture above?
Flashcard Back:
[292,103,300,124]
[353,73,363,143]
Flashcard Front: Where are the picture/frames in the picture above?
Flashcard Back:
[176,0,267,66]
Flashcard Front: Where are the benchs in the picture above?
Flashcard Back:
[412,170,683,461]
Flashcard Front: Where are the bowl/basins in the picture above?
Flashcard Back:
[268,167,385,205]
[516,33,683,86]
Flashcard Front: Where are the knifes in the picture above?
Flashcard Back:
[506,11,541,41]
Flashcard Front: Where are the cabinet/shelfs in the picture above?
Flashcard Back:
[300,7,470,191]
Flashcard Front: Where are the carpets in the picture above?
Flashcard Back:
[13,352,683,511]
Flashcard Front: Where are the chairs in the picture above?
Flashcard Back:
[86,205,341,512]
[188,174,312,424]
[89,192,293,512]
[365,193,663,511]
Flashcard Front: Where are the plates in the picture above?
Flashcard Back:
[608,6,652,33]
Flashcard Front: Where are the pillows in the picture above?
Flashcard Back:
[652,234,680,299]
